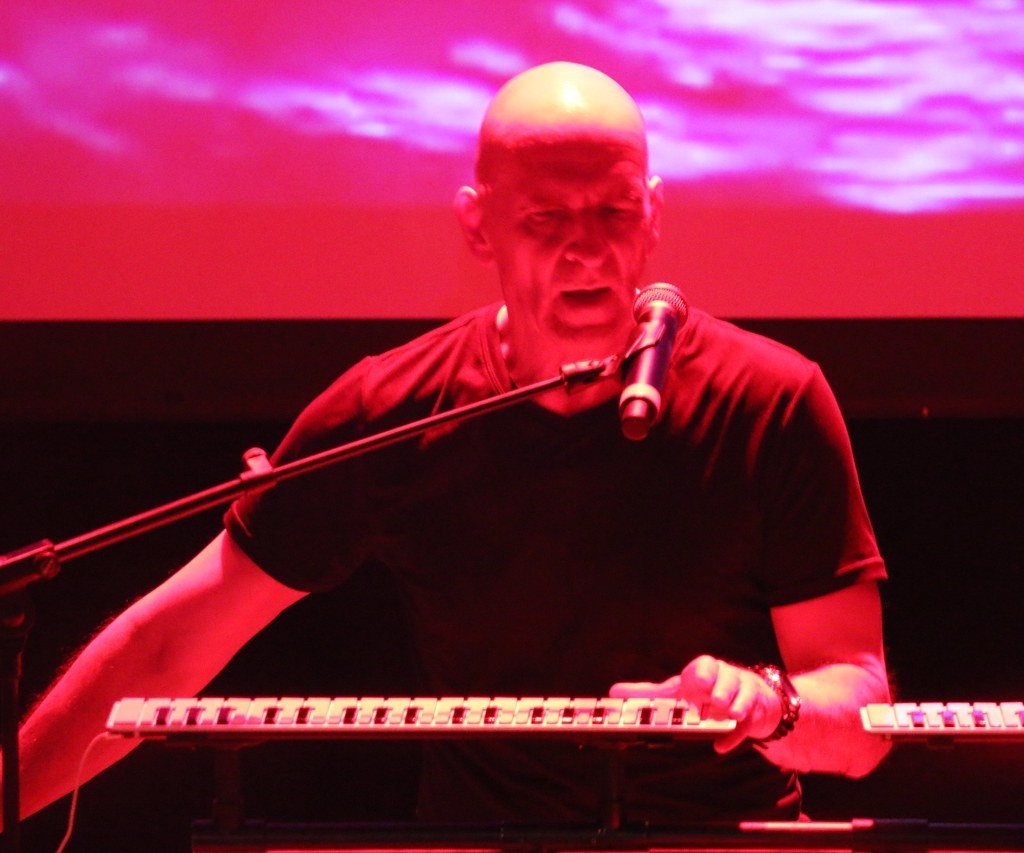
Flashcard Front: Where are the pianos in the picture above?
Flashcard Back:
[101,693,1023,836]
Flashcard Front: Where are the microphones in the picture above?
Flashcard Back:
[617,283,688,440]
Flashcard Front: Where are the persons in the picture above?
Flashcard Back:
[17,61,894,852]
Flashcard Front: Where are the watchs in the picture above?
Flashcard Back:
[744,662,801,753]
